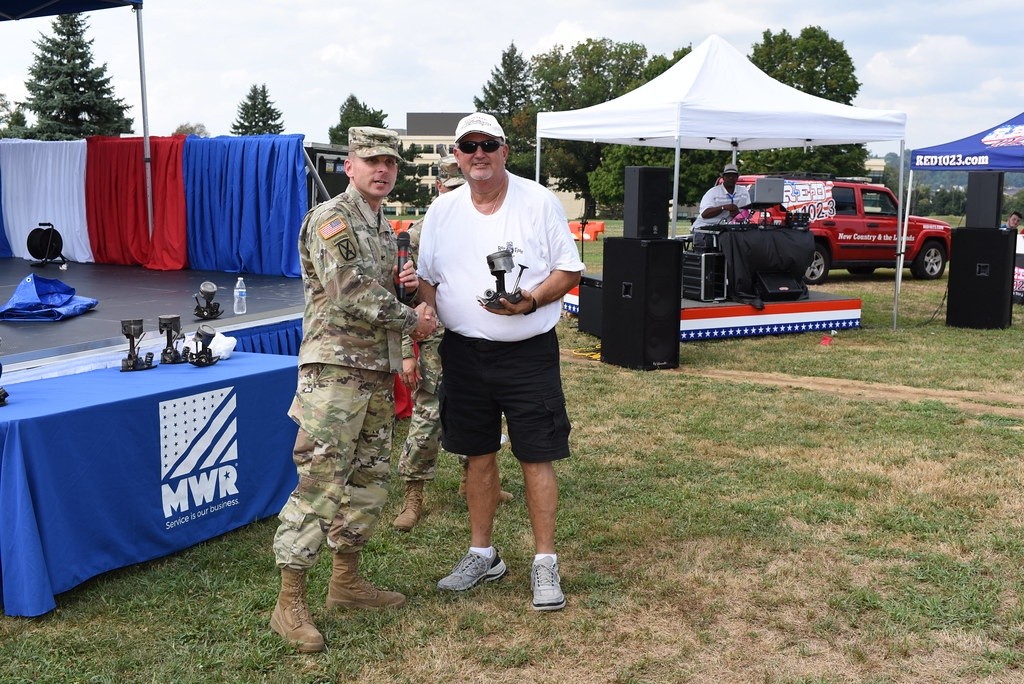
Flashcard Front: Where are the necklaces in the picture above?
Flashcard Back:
[486,190,502,215]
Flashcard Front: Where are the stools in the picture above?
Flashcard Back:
[675,234,693,250]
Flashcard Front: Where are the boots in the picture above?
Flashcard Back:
[393,481,425,530]
[326,553,406,610]
[270,568,324,652]
[457,468,514,502]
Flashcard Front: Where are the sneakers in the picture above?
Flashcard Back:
[530,555,566,612]
[437,546,507,591]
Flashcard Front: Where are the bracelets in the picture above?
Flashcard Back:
[721,206,724,210]
[523,296,537,316]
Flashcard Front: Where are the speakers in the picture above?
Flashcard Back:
[578,165,684,370]
[946,170,1019,331]
[753,270,804,302]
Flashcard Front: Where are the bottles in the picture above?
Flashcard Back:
[234,277,246,314]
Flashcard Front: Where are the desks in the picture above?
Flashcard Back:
[0,353,299,615]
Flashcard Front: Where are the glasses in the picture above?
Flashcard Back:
[456,139,505,154]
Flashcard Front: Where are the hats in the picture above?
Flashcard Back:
[437,154,467,188]
[723,164,739,174]
[348,126,403,161]
[453,112,507,142]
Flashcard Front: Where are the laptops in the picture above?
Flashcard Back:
[740,178,785,209]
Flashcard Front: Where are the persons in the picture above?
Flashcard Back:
[1001,210,1022,228]
[689,164,752,234]
[408,112,587,612]
[268,127,437,653]
[393,154,514,532]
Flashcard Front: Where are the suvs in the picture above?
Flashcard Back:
[715,171,953,285]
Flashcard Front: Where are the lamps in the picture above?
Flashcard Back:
[158,314,190,363]
[192,281,224,320]
[190,324,220,366]
[121,318,157,371]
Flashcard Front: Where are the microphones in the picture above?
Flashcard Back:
[396,232,410,302]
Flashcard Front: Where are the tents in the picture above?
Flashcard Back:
[535,33,908,333]
[898,111,1024,293]
[0,0,154,242]
